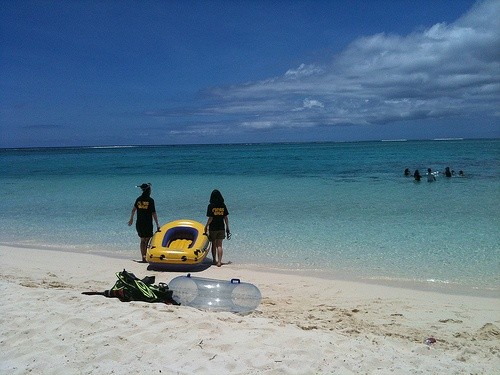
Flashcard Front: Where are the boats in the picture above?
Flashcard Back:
[146,219,211,271]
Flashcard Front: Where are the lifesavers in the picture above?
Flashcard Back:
[168,274,260,311]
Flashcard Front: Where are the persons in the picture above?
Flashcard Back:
[127,184,160,263]
[204,189,231,267]
[403,167,465,183]
